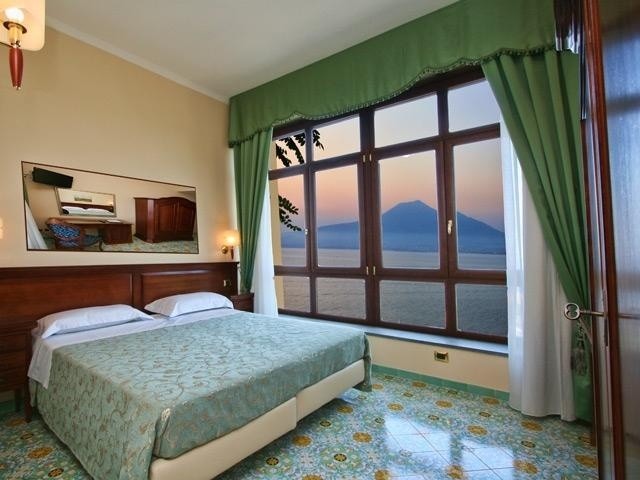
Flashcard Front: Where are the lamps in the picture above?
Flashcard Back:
[220,227,240,256]
[0,1,49,95]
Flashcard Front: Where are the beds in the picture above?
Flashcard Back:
[1,261,372,480]
[61,202,115,216]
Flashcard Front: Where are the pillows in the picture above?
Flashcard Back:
[143,291,236,317]
[63,205,84,211]
[35,302,154,337]
[87,208,108,213]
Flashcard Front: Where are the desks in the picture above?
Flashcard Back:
[49,215,134,244]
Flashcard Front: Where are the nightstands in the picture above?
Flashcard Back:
[228,292,255,312]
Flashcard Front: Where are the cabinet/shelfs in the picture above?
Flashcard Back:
[549,0,638,478]
[133,196,197,243]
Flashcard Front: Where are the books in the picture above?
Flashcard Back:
[106,218,121,223]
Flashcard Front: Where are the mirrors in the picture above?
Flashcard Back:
[21,160,200,255]
[53,186,117,218]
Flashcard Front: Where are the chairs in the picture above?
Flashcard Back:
[44,217,103,252]
